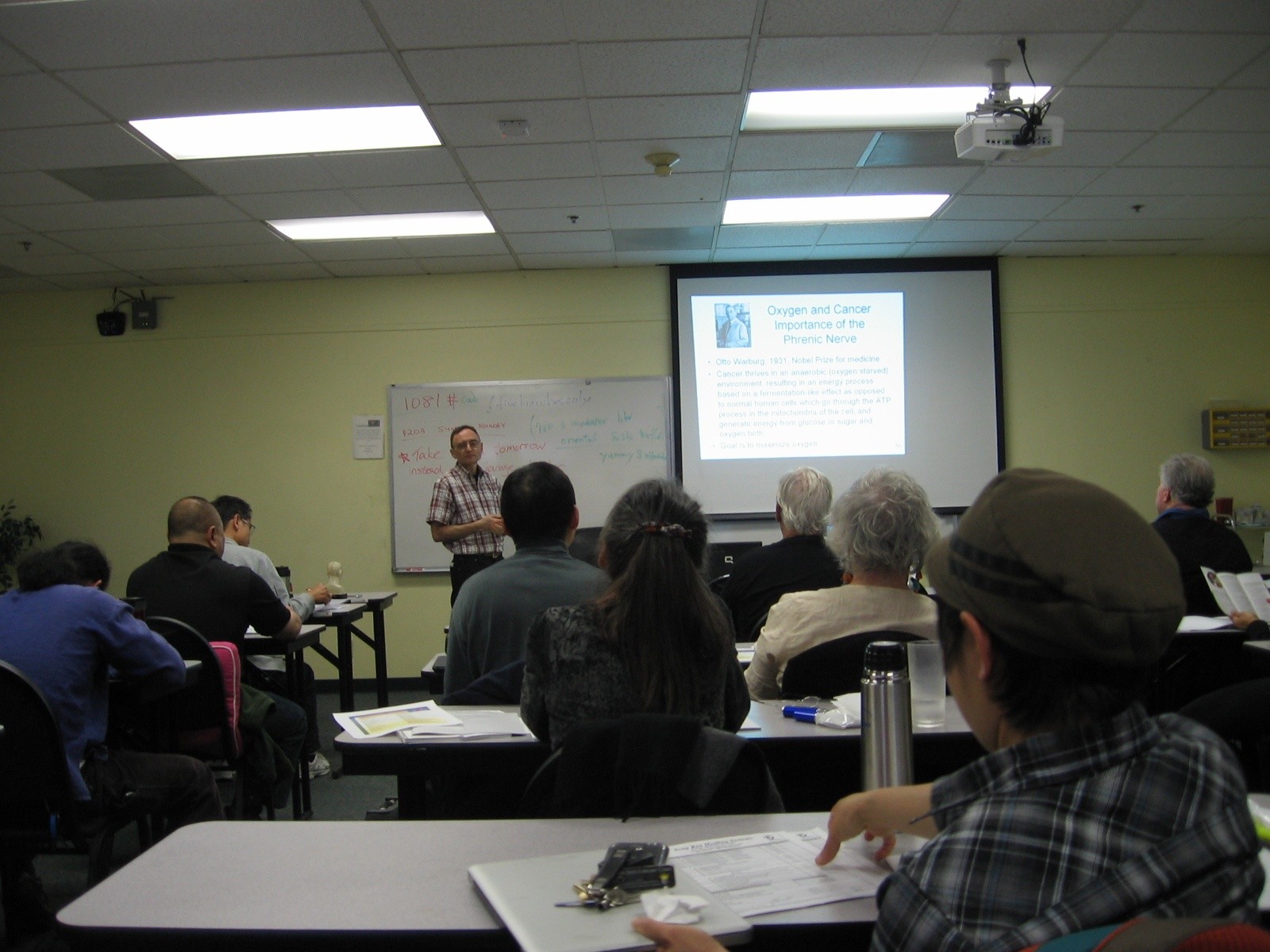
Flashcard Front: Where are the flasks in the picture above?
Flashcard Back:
[119,597,145,621]
[860,641,915,790]
[276,566,290,594]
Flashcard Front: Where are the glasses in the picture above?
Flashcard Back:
[240,517,255,534]
[453,439,482,450]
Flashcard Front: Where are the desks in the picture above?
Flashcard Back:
[332,701,983,811]
[216,590,399,819]
[50,811,943,952]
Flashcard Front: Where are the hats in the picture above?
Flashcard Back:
[923,467,1186,659]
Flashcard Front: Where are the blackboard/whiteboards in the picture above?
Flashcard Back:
[391,376,681,576]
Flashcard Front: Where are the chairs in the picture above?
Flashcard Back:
[135,613,304,849]
[0,655,161,951]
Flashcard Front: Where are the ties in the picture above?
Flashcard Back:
[727,322,732,334]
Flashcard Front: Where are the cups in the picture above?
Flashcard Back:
[1216,496,1232,524]
[908,640,946,727]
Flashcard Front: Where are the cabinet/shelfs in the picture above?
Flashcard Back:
[1212,512,1270,571]
[1201,404,1269,451]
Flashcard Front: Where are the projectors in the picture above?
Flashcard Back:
[953,113,1064,164]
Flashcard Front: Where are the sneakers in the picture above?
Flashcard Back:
[298,754,332,779]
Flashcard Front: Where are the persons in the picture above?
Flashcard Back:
[2,538,238,853]
[123,494,308,825]
[428,422,509,608]
[735,467,942,704]
[724,468,851,644]
[438,456,619,705]
[1142,456,1253,621]
[517,479,751,750]
[1175,611,1270,794]
[630,467,1262,950]
[715,306,748,348]
[213,493,345,781]
